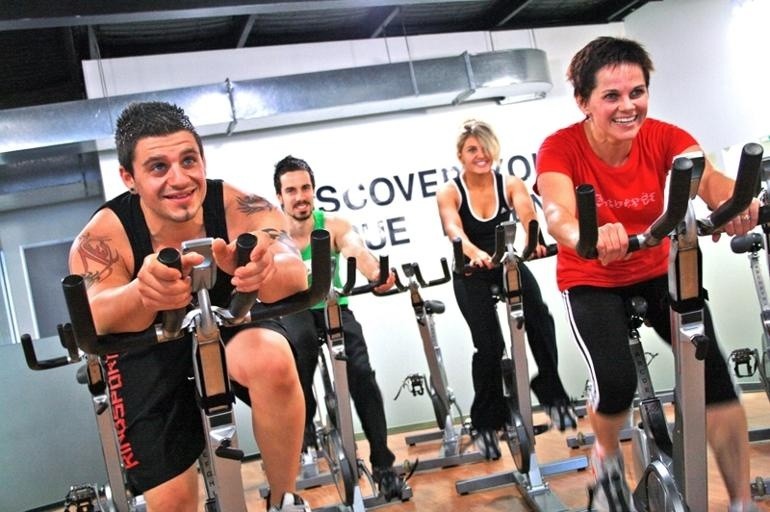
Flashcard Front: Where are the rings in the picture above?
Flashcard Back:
[741,213,750,221]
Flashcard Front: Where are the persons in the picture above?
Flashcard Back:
[532,35,761,512]
[68,101,317,512]
[272,153,397,490]
[435,117,580,432]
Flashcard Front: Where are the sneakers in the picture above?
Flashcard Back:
[590,437,630,512]
[372,463,402,503]
[301,422,318,453]
[469,425,501,459]
[545,398,579,431]
